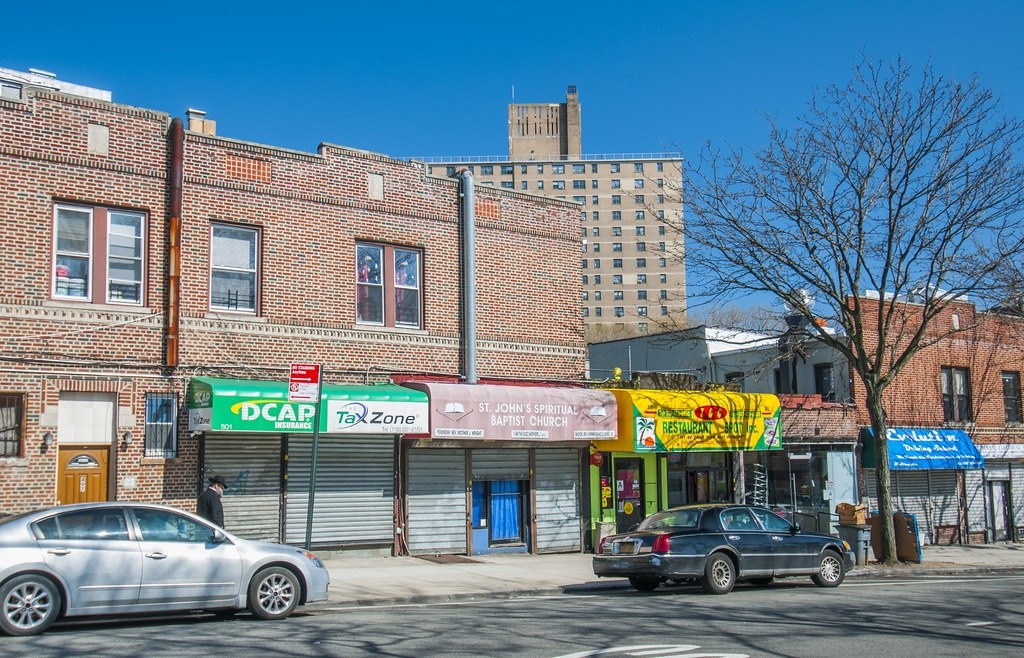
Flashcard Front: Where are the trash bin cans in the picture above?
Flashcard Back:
[832,524,872,566]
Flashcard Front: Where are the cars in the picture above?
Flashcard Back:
[592,503,857,594]
[0,501,332,637]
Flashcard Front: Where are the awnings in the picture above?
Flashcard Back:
[861,426,984,470]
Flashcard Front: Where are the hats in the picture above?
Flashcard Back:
[208,476,228,489]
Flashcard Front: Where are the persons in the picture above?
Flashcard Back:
[196,475,229,528]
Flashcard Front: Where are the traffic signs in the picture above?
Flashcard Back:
[287,363,321,405]
